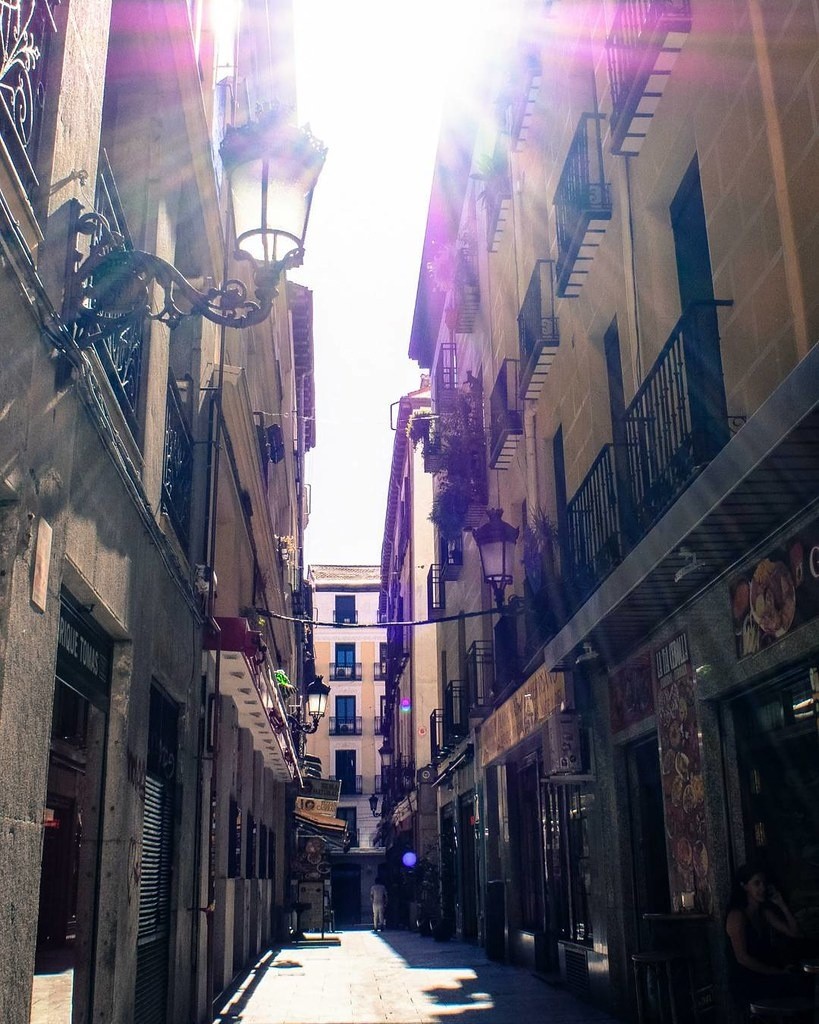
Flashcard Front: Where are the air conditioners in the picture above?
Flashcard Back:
[541,714,583,776]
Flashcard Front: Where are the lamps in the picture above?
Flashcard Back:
[217,97,328,305]
[305,674,331,726]
[472,507,519,613]
[369,793,378,812]
[378,741,395,772]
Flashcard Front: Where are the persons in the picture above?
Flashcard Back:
[370,878,388,932]
[725,862,819,1024]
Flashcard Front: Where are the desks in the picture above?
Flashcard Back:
[642,911,716,1024]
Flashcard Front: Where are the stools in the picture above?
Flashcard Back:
[631,952,684,1024]
